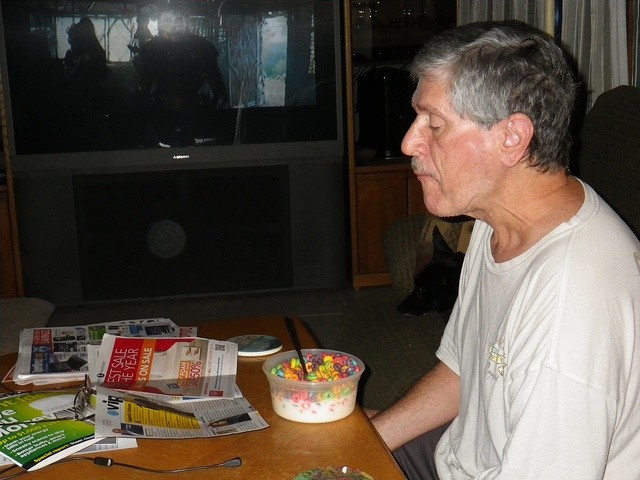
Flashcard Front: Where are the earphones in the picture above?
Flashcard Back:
[220,457,241,468]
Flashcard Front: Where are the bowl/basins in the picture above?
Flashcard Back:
[261,347,365,424]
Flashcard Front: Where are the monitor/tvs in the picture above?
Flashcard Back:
[0,0,344,178]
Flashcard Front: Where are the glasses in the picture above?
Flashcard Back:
[73,373,97,421]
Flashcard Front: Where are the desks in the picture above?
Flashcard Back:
[1,312,407,480]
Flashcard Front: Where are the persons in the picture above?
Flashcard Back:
[362,19,639,480]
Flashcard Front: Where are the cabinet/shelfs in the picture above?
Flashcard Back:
[355,169,428,286]
